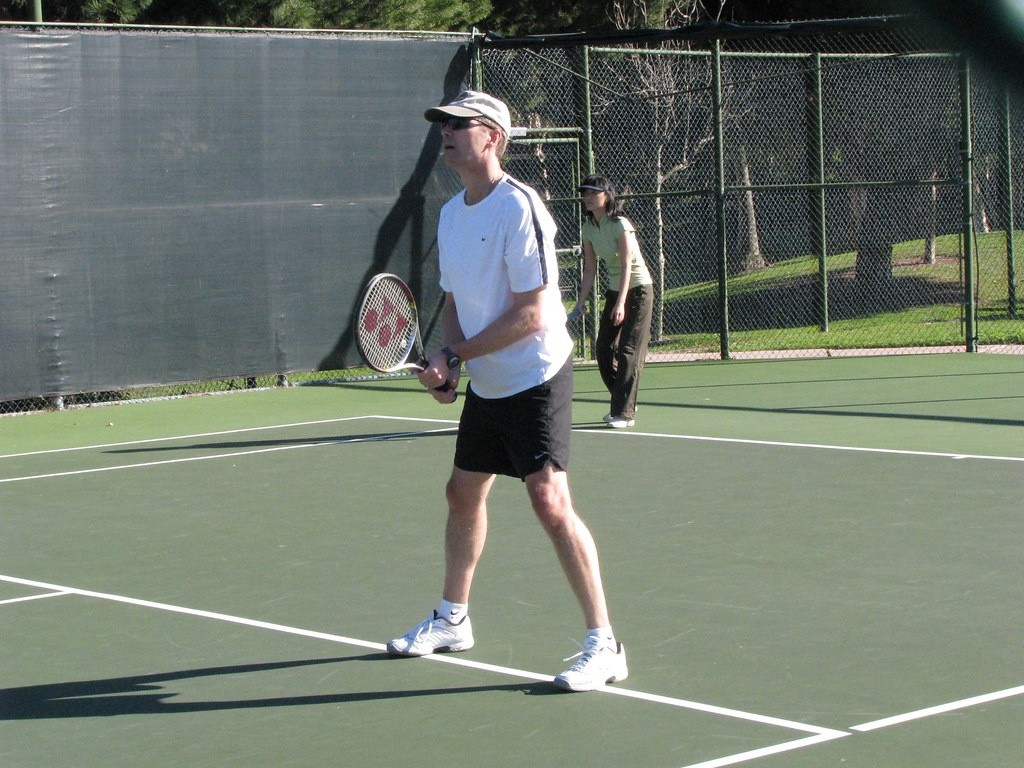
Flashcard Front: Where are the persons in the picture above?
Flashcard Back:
[387,89,628,692]
[572,174,654,428]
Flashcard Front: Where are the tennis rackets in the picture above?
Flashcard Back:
[352,271,458,403]
[566,304,587,319]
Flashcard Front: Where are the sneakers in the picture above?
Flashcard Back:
[386,610,475,656]
[553,636,629,691]
[603,405,638,421]
[607,416,635,428]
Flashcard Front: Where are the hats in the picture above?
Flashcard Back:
[424,90,511,137]
[577,183,613,193]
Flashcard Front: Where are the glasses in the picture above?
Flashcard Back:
[441,115,483,131]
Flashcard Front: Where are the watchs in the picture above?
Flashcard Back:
[441,346,463,370]
